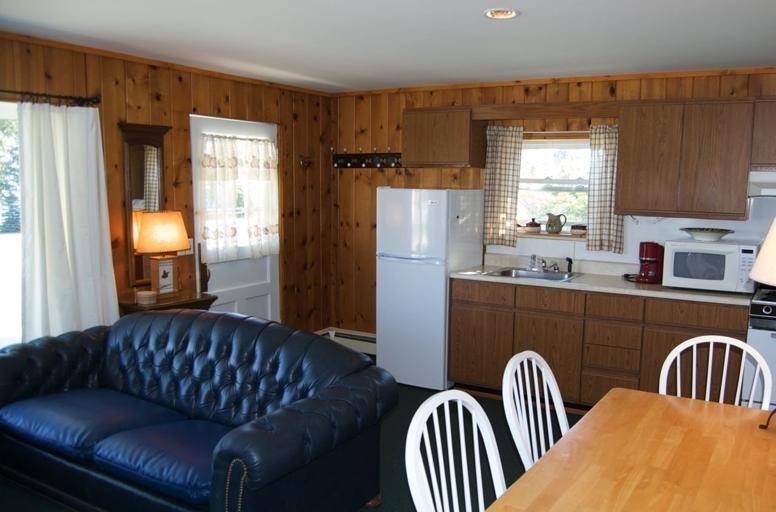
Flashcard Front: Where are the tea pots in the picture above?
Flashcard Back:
[545,211,567,234]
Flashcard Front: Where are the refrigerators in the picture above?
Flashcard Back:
[374,185,484,392]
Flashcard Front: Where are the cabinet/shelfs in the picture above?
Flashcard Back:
[752,96,775,168]
[400,108,486,167]
[448,277,515,409]
[582,290,646,415]
[514,283,585,416]
[613,97,754,223]
[638,294,751,407]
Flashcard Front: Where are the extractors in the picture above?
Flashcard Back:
[745,169,776,198]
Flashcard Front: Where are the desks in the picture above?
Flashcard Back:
[117,292,218,315]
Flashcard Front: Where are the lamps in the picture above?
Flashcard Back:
[133,212,191,303]
[748,217,775,430]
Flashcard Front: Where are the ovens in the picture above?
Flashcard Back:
[741,317,776,410]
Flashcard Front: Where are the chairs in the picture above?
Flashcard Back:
[403,390,509,511]
[503,349,577,472]
[658,335,771,411]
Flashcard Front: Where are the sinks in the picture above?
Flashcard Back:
[483,267,584,283]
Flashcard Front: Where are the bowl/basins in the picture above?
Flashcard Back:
[136,290,157,305]
[570,224,587,235]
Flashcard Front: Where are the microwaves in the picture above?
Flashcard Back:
[661,238,764,294]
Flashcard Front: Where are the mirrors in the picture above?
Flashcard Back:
[117,121,172,288]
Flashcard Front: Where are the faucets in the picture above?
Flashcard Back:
[536,256,549,273]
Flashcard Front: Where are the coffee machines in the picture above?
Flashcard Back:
[635,241,663,284]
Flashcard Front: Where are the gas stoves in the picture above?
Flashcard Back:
[750,289,776,317]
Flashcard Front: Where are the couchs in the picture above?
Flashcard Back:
[0,307,397,512]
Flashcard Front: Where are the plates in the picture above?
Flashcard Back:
[679,226,735,242]
